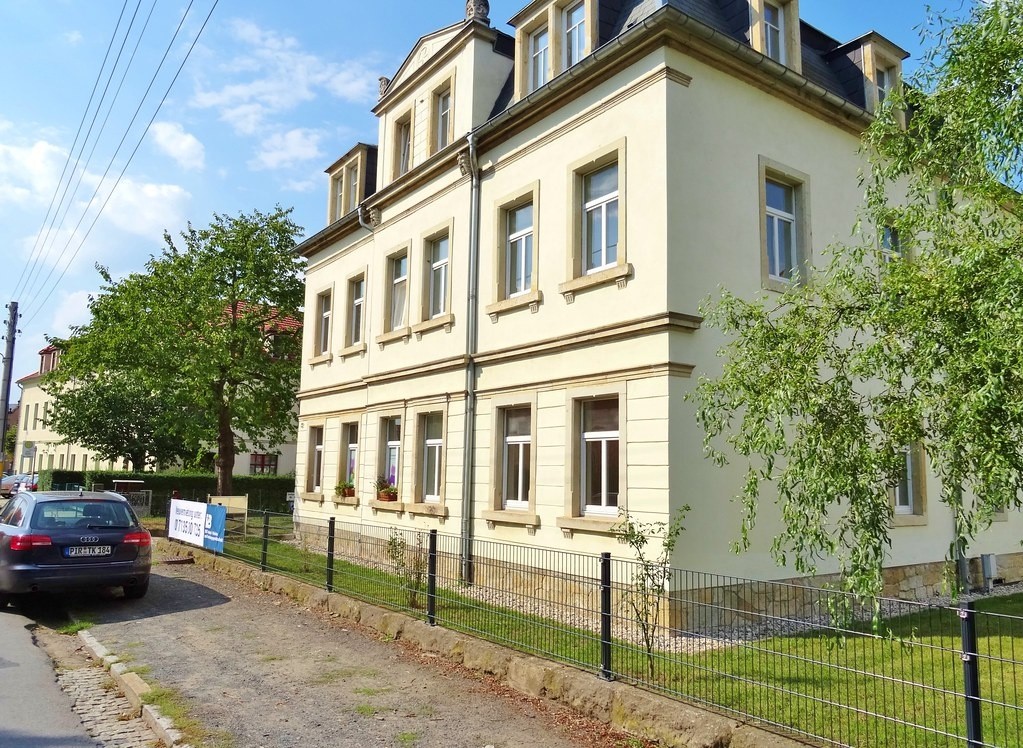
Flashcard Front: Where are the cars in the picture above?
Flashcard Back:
[0,491,153,606]
[1,472,39,498]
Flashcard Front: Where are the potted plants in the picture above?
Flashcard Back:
[376,475,397,501]
[334,480,355,497]
